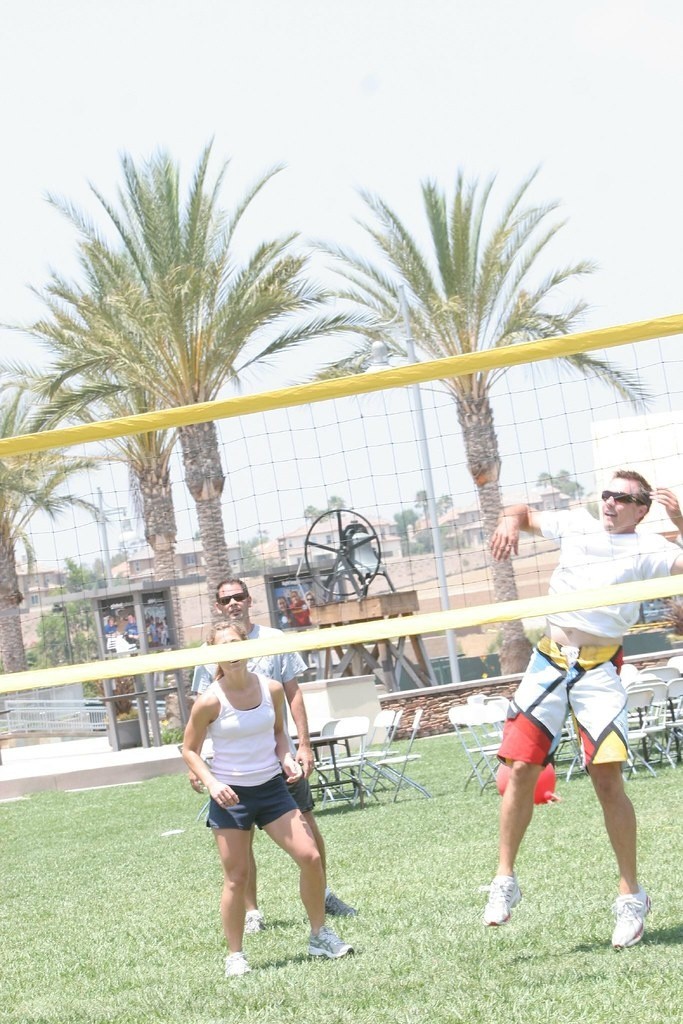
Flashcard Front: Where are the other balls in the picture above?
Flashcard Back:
[496,762,557,805]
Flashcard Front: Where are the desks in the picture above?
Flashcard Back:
[292,732,365,797]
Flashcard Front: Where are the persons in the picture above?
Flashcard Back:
[182,624,355,978]
[482,469,683,947]
[104,613,139,652]
[145,614,170,646]
[277,591,315,628]
[191,578,358,934]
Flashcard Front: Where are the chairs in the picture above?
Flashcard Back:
[290,655,683,810]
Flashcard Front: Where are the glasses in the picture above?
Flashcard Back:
[307,599,314,601]
[218,592,247,605]
[602,491,646,505]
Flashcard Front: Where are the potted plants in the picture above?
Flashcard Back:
[98,679,142,751]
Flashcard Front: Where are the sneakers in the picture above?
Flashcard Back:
[223,951,251,978]
[609,884,651,950]
[479,872,522,925]
[244,910,266,934]
[309,925,355,959]
[324,892,359,919]
